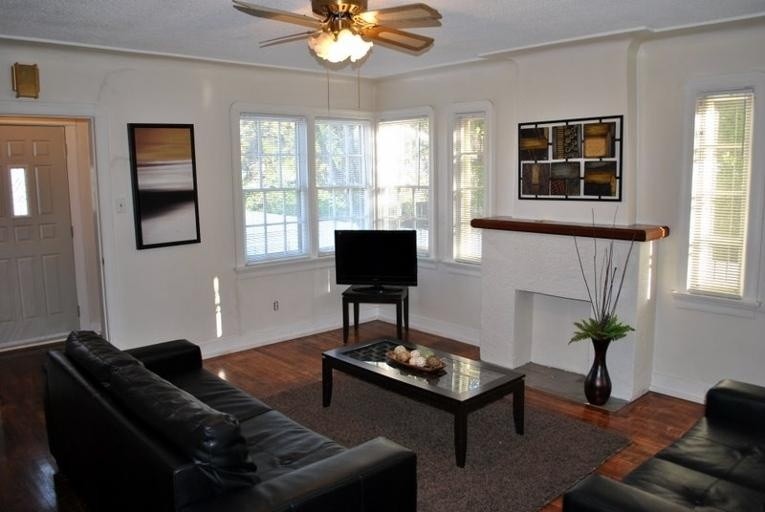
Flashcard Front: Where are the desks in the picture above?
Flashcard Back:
[341,285,409,344]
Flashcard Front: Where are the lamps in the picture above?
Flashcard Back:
[306,24,374,64]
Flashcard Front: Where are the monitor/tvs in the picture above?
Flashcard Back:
[334,229,417,294]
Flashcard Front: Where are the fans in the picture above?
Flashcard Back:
[228,0,442,54]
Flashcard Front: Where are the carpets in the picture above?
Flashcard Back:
[259,370,633,511]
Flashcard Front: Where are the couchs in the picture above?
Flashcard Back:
[44,328,418,511]
[560,378,765,511]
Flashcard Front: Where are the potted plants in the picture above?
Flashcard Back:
[565,204,638,406]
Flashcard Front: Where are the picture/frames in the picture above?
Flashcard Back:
[125,121,201,250]
